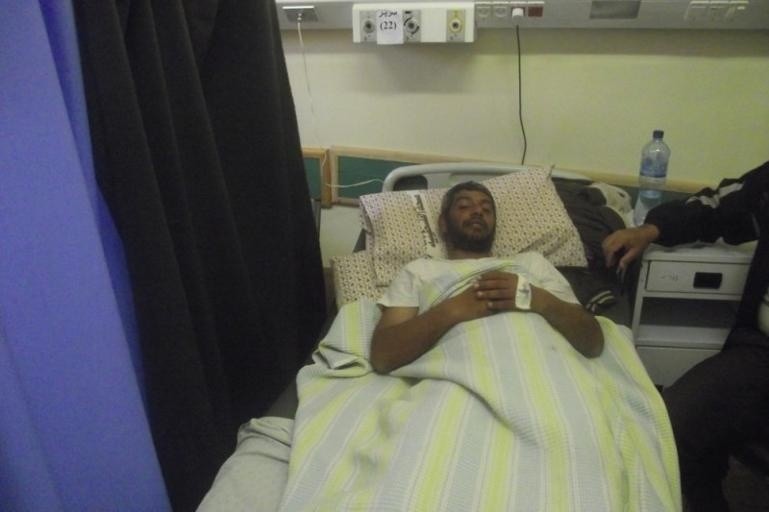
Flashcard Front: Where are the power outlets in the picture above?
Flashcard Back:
[476,2,527,30]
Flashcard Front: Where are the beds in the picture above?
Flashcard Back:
[169,164,637,510]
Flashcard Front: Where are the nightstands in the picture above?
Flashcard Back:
[634,239,760,400]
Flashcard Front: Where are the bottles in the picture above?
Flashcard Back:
[632,130,671,228]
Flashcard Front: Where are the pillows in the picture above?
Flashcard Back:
[328,166,589,313]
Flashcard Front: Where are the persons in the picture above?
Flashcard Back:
[368,180,604,376]
[601,161,768,511]
